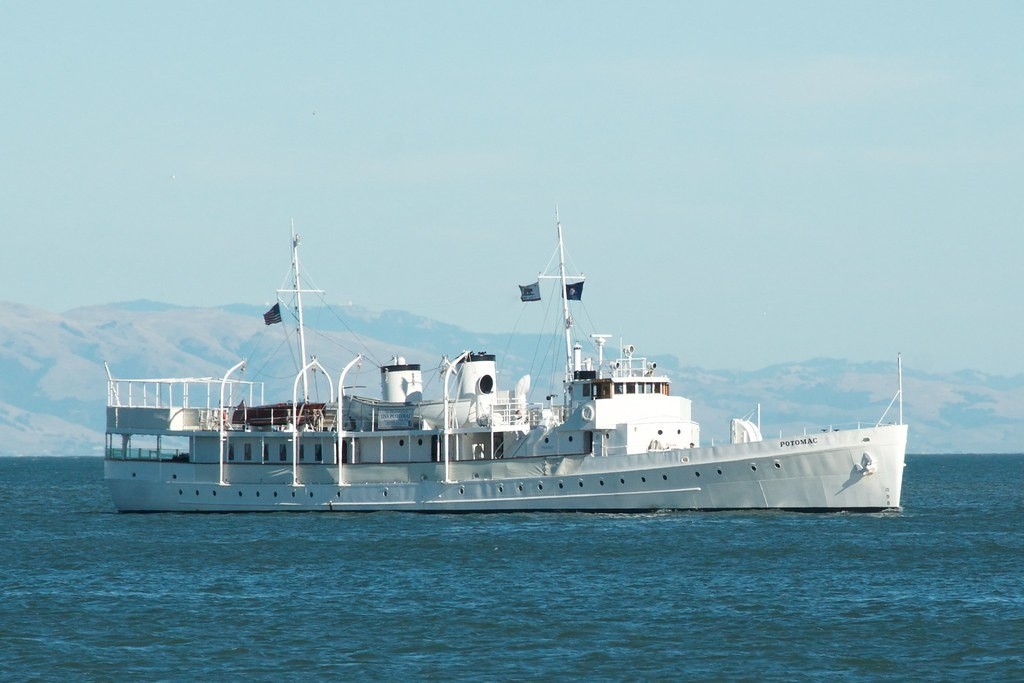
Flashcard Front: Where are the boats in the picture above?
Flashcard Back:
[102,205,908,514]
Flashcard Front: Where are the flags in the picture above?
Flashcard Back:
[519,281,541,301]
[263,303,282,325]
[562,282,583,300]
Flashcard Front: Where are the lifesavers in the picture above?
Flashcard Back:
[581,404,594,421]
